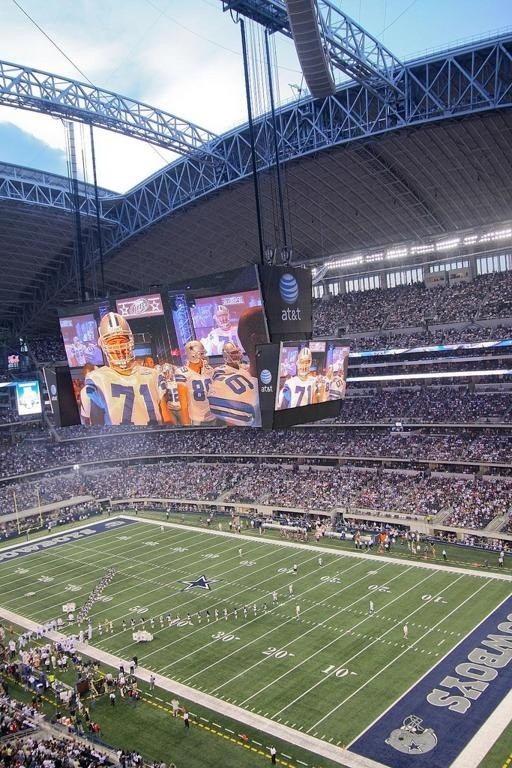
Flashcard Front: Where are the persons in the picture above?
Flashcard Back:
[0,269,512,768]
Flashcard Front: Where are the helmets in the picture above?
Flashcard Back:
[222,340,243,365]
[155,363,174,382]
[296,348,312,376]
[185,341,209,364]
[98,312,137,370]
[213,304,231,330]
